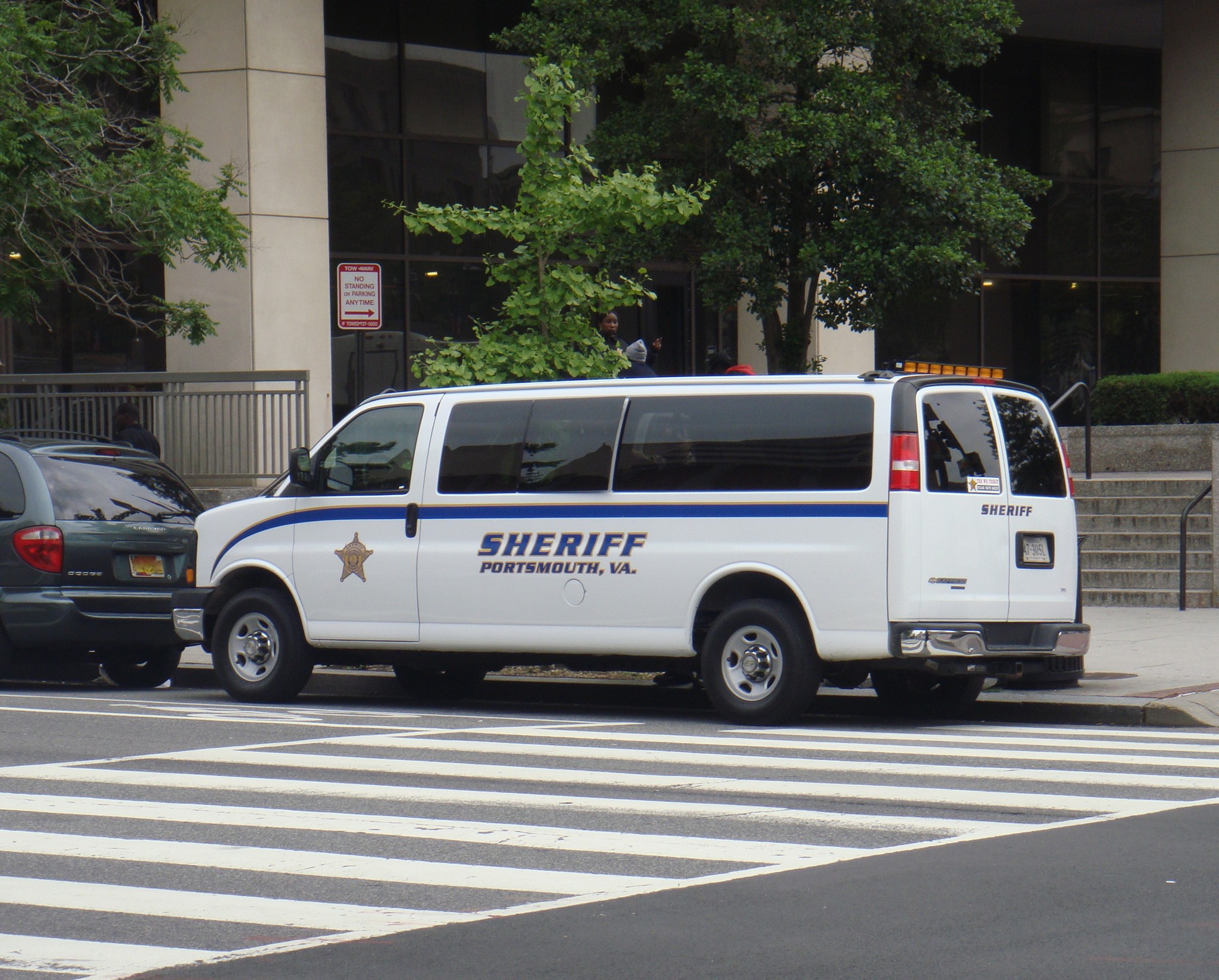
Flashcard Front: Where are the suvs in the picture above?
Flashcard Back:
[0,429,207,689]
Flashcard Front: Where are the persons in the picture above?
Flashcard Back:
[637,416,697,464]
[114,403,159,457]
[721,362,756,376]
[592,310,662,367]
[617,340,660,379]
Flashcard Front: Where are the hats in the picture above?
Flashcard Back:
[625,340,646,363]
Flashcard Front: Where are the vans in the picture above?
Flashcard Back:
[171,362,1092,726]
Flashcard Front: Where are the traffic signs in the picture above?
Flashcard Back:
[337,264,383,332]
